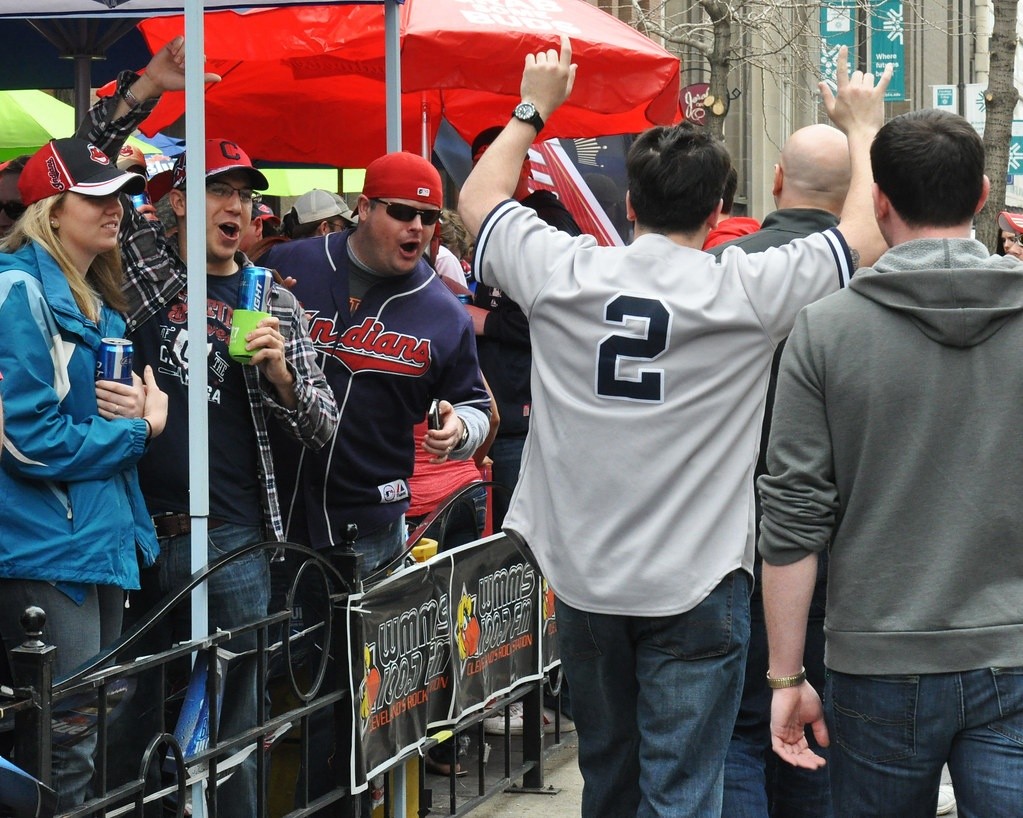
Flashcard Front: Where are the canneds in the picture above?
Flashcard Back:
[94,338,133,380]
[130,194,151,211]
[455,294,474,305]
[237,265,274,313]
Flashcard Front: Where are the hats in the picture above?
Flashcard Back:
[351,149,444,208]
[291,189,360,224]
[250,202,280,227]
[18,135,146,206]
[115,144,147,172]
[471,125,536,182]
[172,137,269,191]
[997,210,1023,234]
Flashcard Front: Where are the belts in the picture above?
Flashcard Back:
[152,511,224,540]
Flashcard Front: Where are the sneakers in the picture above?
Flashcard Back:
[484,701,577,734]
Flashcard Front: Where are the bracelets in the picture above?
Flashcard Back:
[123,82,142,109]
[767,666,806,689]
[458,418,469,448]
[142,418,152,442]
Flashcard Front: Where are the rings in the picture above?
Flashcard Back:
[115,406,119,413]
[448,447,451,451]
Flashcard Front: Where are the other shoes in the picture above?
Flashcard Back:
[936,785,957,814]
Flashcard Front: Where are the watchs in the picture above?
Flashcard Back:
[511,102,545,136]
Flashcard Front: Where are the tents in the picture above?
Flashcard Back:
[0,0,680,664]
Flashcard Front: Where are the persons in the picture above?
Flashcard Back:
[997,210,1023,264]
[695,123,853,818]
[238,186,359,268]
[421,118,584,774]
[116,145,179,240]
[76,34,340,818]
[757,108,1023,818]
[259,151,490,818]
[0,155,35,239]
[0,138,165,818]
[457,26,897,818]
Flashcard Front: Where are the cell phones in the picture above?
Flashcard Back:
[427,399,441,432]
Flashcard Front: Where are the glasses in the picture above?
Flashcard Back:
[0,199,26,222]
[372,198,444,226]
[206,182,264,203]
